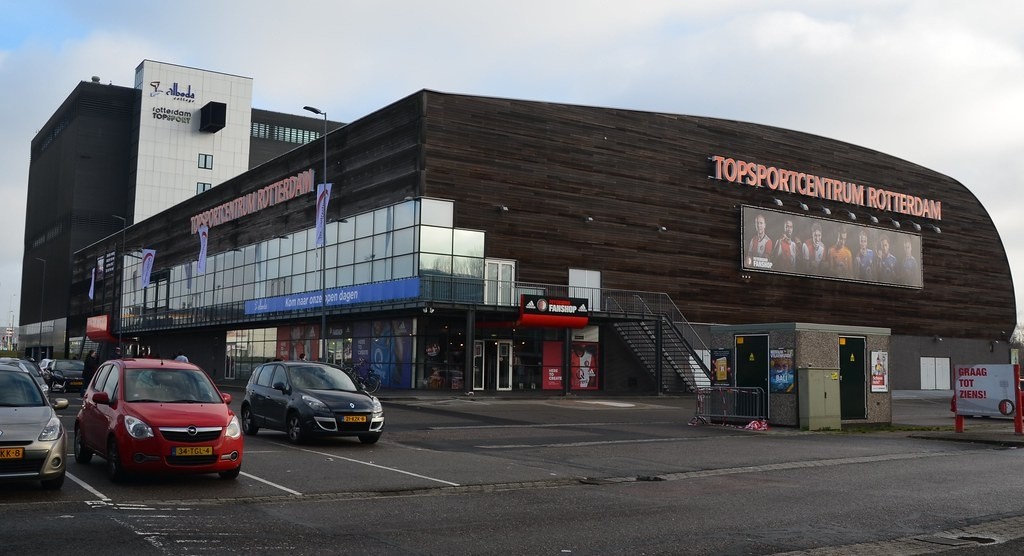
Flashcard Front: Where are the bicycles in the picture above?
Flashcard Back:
[342,363,382,394]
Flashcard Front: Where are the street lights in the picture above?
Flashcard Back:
[304,105,328,363]
[112,213,126,357]
[35,257,47,362]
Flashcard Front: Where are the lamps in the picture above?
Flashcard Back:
[840,209,856,220]
[990,341,999,344]
[923,224,941,234]
[657,225,666,232]
[499,205,508,211]
[759,195,783,207]
[271,232,288,239]
[791,200,809,211]
[862,212,878,223]
[405,197,419,201]
[934,337,943,342]
[814,204,831,215]
[227,248,241,251]
[585,217,593,222]
[329,217,347,223]
[885,217,900,228]
[905,220,921,231]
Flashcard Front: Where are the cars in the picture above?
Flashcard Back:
[0,363,70,492]
[0,357,85,406]
[240,361,384,445]
[74,357,244,481]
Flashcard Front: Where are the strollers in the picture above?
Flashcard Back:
[45,368,68,393]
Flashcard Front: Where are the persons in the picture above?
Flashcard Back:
[175,350,188,363]
[898,236,921,286]
[79,350,99,397]
[771,358,792,371]
[875,233,898,283]
[828,224,854,279]
[770,218,801,270]
[875,357,882,371]
[113,347,122,360]
[800,220,827,274]
[300,353,307,361]
[367,319,404,389]
[854,230,876,281]
[747,214,773,260]
